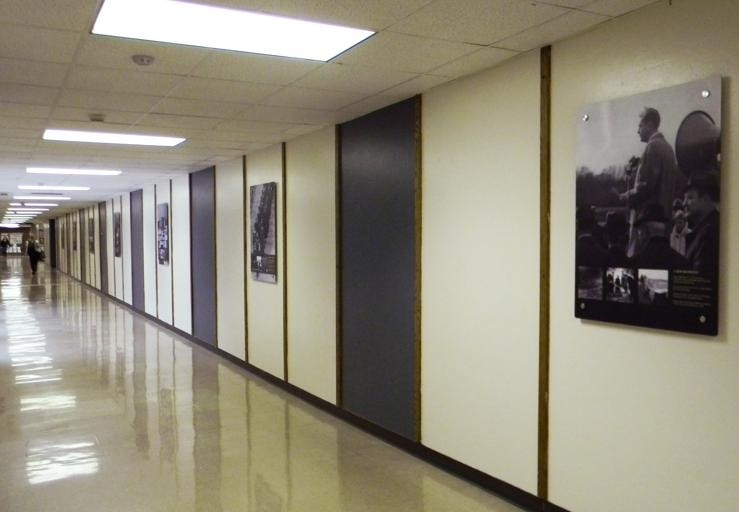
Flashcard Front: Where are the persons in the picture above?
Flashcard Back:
[26,234,38,272]
[577,107,720,301]
[29,274,46,302]
[0,236,12,254]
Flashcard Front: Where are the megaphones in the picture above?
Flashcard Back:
[675,111,721,168]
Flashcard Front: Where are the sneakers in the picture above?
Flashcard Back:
[32,272,35,275]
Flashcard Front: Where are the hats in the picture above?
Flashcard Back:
[680,170,720,195]
